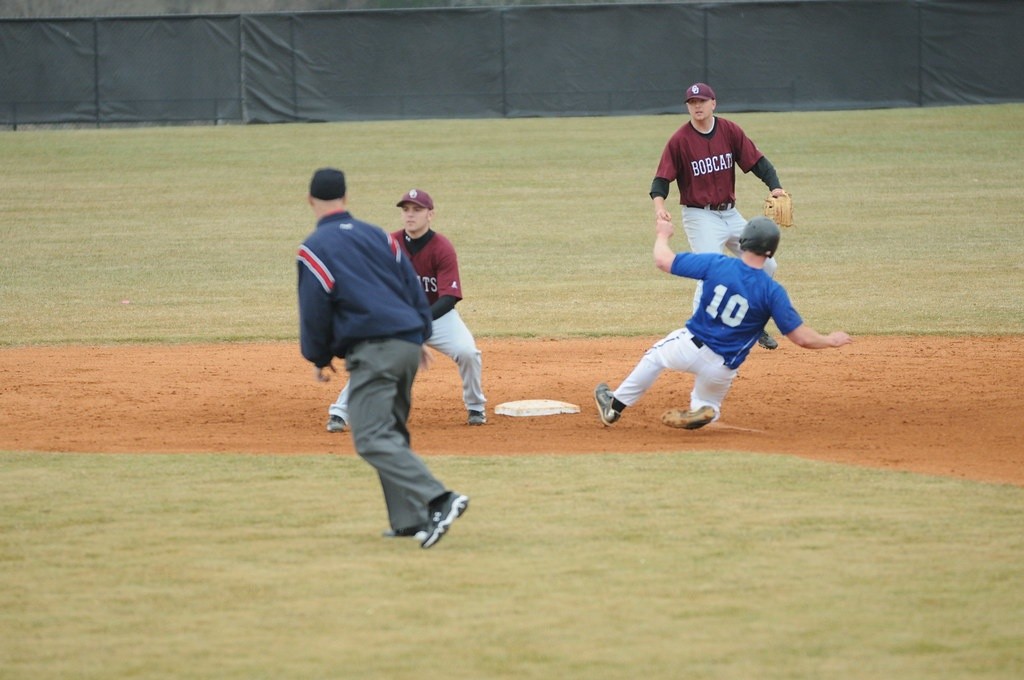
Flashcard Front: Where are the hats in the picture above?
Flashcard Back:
[311,169,345,199]
[684,83,716,102]
[739,217,779,258]
[396,189,434,209]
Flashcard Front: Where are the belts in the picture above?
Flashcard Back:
[692,337,703,349]
[686,202,735,210]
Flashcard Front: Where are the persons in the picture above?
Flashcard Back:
[296,169,469,553]
[595,216,855,430]
[649,83,793,351]
[326,187,485,426]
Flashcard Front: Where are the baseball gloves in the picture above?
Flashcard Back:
[763,189,795,228]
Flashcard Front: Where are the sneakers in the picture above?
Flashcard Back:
[326,414,346,432]
[757,330,778,350]
[468,409,486,425]
[421,491,468,549]
[594,383,621,426]
[659,404,715,429]
[382,523,427,539]
